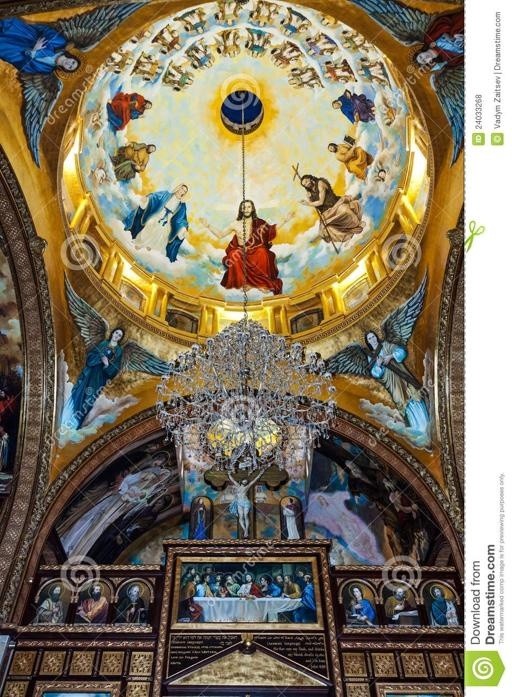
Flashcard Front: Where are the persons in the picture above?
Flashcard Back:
[0,2,149,171]
[282,457,432,565]
[57,450,172,563]
[74,583,111,625]
[177,566,317,623]
[429,584,460,626]
[347,584,377,626]
[319,265,430,432]
[384,584,414,624]
[227,469,268,541]
[59,269,191,432]
[115,584,149,622]
[351,0,464,167]
[29,584,64,627]
[105,0,395,297]
[192,497,212,540]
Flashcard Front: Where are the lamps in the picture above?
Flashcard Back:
[149,92,340,484]
